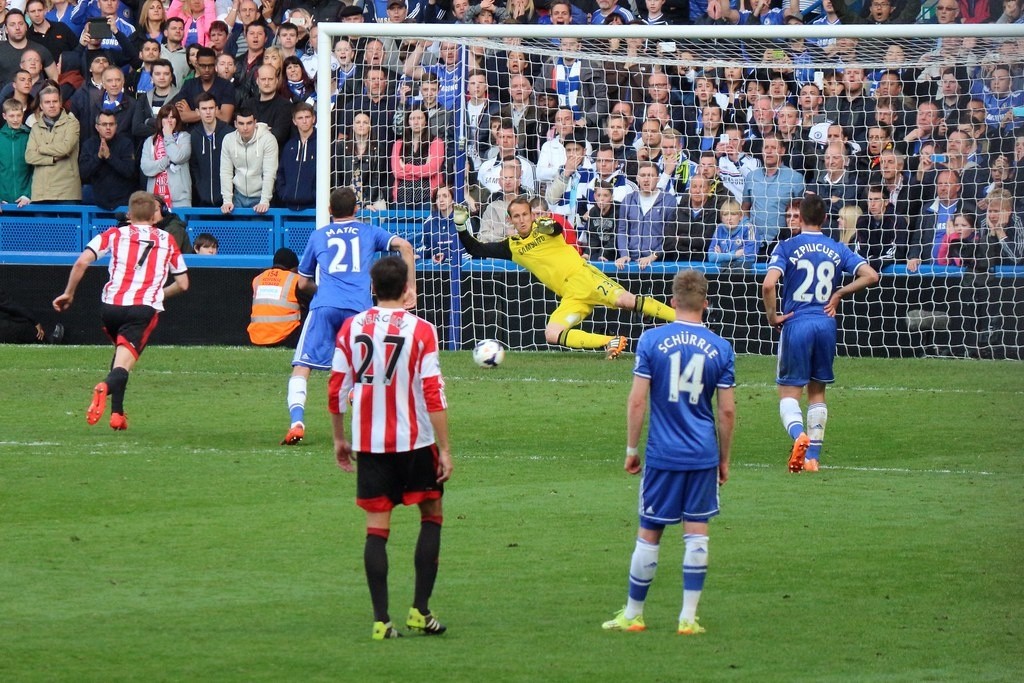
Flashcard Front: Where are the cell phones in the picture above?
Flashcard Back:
[814,72,824,90]
[658,41,676,52]
[290,17,305,26]
[87,17,113,39]
[769,50,784,59]
[930,155,947,163]
[720,134,729,145]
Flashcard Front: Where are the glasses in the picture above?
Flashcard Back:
[968,108,984,112]
[649,83,667,88]
[99,122,115,127]
[937,5,956,11]
[873,2,891,7]
[198,64,214,68]
[22,59,40,63]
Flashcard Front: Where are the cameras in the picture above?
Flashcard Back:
[989,54,1002,62]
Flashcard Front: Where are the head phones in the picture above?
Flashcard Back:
[153,197,169,217]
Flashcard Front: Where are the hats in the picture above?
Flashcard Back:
[340,5,364,16]
[387,0,407,10]
[88,51,111,69]
[540,88,559,105]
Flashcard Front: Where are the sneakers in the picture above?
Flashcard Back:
[372,621,403,640]
[805,459,820,472]
[406,607,447,635]
[606,335,629,360]
[602,605,646,632]
[788,433,810,473]
[86,382,108,425]
[678,618,707,635]
[109,413,127,430]
[281,424,305,446]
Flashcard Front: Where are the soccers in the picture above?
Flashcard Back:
[472,340,506,369]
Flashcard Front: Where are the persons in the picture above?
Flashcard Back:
[601,269,736,635]
[327,257,454,640]
[452,198,676,361]
[0,0,1024,360]
[762,195,879,473]
[53,191,189,430]
[247,247,318,348]
[280,187,416,445]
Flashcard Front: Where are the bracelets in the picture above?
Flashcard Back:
[626,446,638,456]
